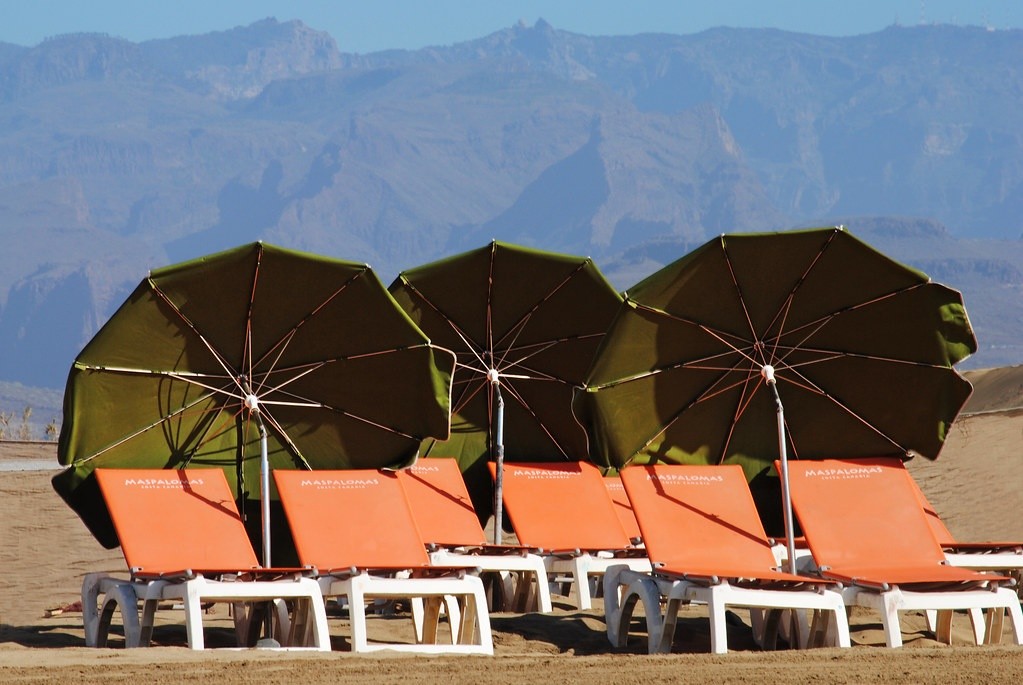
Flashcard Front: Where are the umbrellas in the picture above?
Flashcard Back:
[580,225,978,583]
[50,239,456,640]
[386,240,625,614]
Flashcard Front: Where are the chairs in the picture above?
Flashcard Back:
[82,456,1023,657]
